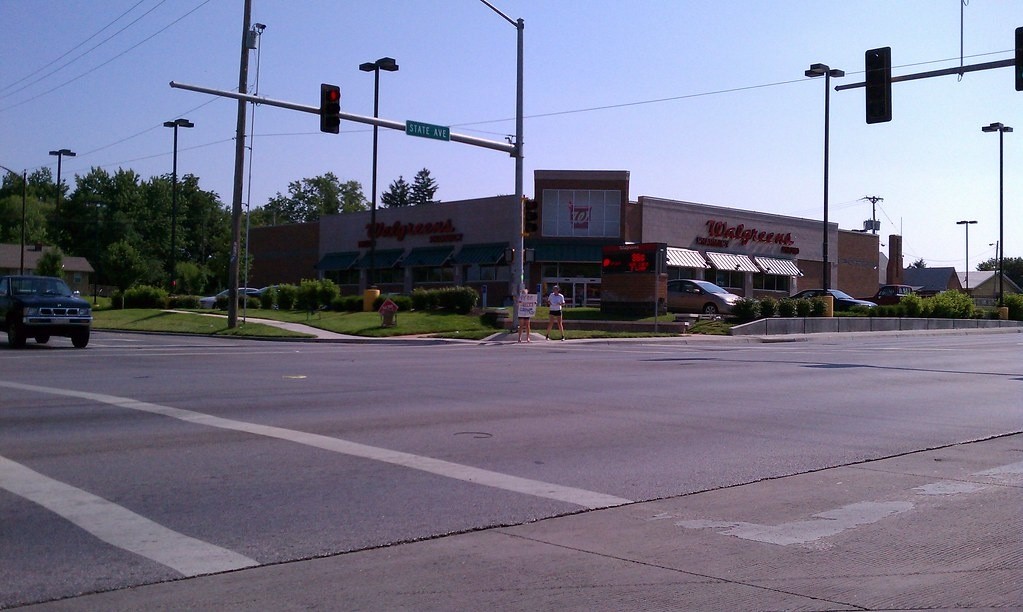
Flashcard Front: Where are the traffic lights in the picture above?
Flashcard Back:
[504,248,515,263]
[319,83,342,134]
[524,248,535,264]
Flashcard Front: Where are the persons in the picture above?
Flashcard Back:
[546,286,566,342]
[516,288,537,343]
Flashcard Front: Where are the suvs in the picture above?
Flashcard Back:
[0,273,95,351]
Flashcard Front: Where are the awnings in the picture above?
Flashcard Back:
[400,246,455,267]
[754,256,804,277]
[523,239,625,263]
[313,252,360,271]
[665,247,711,269]
[450,243,508,265]
[354,249,404,269]
[706,252,761,272]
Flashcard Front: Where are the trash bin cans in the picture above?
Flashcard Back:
[363,289,380,312]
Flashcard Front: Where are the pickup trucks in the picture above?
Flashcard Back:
[854,283,941,305]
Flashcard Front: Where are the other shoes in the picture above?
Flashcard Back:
[518,340,521,344]
[561,338,564,341]
[527,341,532,343]
[546,338,549,341]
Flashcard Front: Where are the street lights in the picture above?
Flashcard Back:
[981,122,1013,307]
[805,63,845,297]
[987,241,999,300]
[956,220,979,297]
[162,117,194,298]
[48,147,77,246]
[357,56,400,286]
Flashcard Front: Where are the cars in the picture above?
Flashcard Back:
[667,277,747,315]
[778,288,879,313]
[198,284,299,310]
